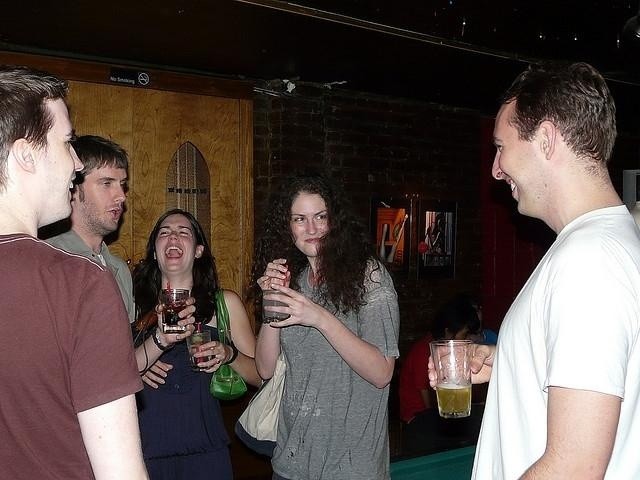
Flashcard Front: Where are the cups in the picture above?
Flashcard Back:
[158,288,190,335]
[261,289,290,320]
[185,328,213,374]
[428,340,474,420]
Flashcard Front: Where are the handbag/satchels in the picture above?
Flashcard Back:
[234,351,286,457]
[209,364,248,400]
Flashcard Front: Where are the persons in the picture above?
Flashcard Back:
[458,294,498,346]
[253,171,404,478]
[426,57,636,478]
[131,208,262,478]
[398,298,483,442]
[39,133,199,380]
[0,63,151,478]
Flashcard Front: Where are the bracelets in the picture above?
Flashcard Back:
[224,343,238,366]
[152,325,176,352]
